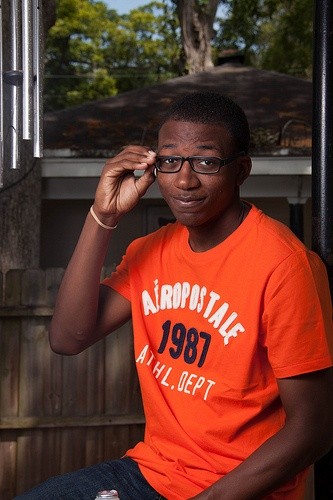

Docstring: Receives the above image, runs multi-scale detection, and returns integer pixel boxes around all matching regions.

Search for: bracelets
[90,203,119,230]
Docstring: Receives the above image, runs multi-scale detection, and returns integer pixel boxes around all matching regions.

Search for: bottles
[94,490,119,500]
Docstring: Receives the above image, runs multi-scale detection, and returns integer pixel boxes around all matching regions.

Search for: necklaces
[238,202,248,226]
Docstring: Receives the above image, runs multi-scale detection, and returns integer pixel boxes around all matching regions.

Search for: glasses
[153,152,244,176]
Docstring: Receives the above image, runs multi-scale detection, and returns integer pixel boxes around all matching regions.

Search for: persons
[7,93,332,500]
